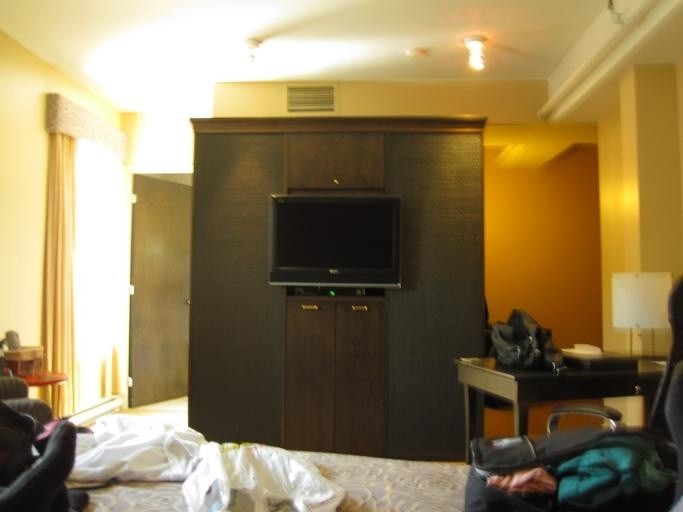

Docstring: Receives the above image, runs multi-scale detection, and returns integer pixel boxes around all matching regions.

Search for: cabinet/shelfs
[281,294,389,457]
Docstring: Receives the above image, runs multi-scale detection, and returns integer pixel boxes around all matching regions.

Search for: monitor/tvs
[267,193,402,290]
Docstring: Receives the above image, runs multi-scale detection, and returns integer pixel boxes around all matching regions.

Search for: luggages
[464,403,679,512]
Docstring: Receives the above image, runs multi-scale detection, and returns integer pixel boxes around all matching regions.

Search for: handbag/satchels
[488,308,562,370]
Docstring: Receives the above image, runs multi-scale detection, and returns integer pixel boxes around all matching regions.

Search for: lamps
[610,271,673,360]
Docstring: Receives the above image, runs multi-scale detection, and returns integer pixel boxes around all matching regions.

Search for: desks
[454,355,669,463]
[8,371,67,386]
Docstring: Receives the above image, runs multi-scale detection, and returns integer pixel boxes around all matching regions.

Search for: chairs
[546,272,683,439]
